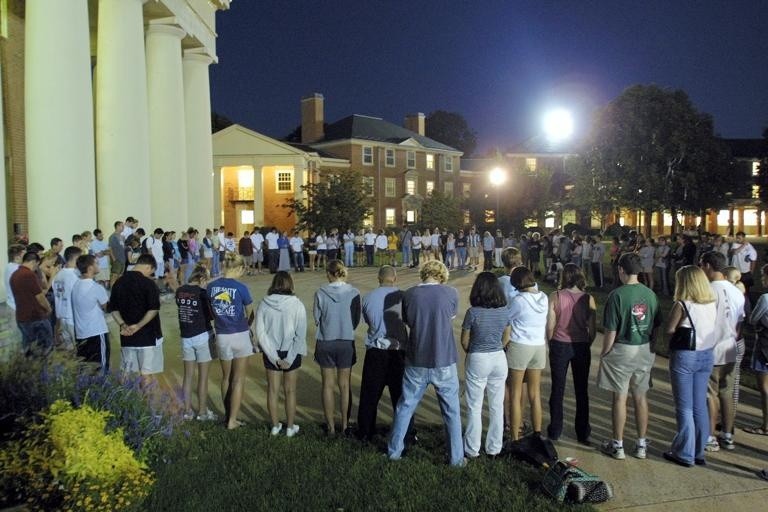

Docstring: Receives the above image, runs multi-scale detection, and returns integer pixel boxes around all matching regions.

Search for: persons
[501,267,548,441]
[459,272,512,459]
[547,263,597,447]
[387,260,468,470]
[356,264,418,445]
[700,252,736,452]
[597,253,660,459]
[251,271,306,438]
[665,264,717,467]
[8,215,767,434]
[313,260,360,436]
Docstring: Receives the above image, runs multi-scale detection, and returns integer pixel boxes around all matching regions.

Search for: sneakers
[183,412,418,462]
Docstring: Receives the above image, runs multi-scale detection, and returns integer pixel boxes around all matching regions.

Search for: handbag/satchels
[573,294,596,342]
[670,327,696,350]
